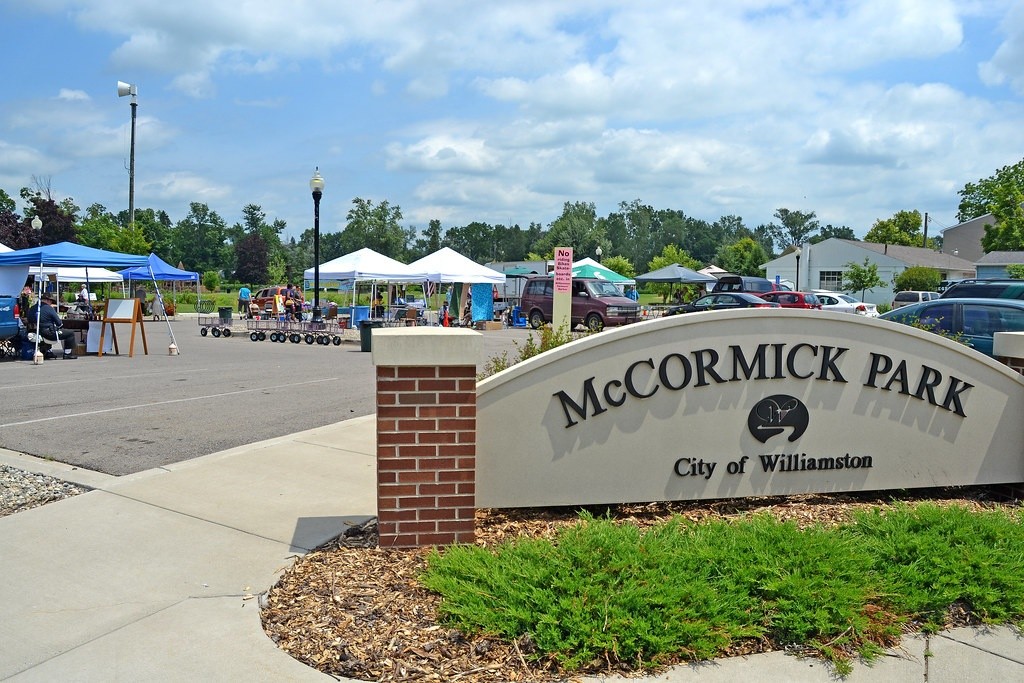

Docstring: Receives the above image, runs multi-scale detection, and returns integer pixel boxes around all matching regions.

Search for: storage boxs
[476,321,504,330]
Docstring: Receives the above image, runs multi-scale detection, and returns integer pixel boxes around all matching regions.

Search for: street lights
[30,213,42,246]
[596,243,602,263]
[116,81,138,237]
[794,245,801,291]
[310,166,323,313]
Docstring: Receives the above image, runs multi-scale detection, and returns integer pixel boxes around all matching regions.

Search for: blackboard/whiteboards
[102,298,142,323]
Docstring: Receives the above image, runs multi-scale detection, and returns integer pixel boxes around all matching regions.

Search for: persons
[21,284,32,310]
[625,285,639,301]
[674,286,706,304]
[439,300,450,327]
[152,293,164,321]
[372,295,384,317]
[59,296,68,312]
[30,292,78,359]
[136,288,147,314]
[81,284,88,299]
[237,283,251,320]
[76,294,86,302]
[286,283,305,322]
[460,302,472,327]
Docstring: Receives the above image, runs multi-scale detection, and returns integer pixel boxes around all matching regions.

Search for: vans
[249,287,304,315]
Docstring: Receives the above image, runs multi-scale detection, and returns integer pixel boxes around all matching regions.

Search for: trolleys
[248,319,345,346]
[196,316,234,337]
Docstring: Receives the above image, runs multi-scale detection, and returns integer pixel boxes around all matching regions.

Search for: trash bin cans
[359,320,382,352]
[218,306,233,325]
[512,306,526,327]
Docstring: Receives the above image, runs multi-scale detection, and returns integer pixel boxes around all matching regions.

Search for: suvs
[521,276,643,332]
[875,277,1024,365]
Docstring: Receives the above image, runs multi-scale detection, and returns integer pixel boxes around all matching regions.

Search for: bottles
[168,341,177,355]
[33,347,43,365]
[67,306,102,321]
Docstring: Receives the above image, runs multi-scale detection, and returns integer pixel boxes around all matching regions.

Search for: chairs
[27,316,58,360]
[374,306,423,328]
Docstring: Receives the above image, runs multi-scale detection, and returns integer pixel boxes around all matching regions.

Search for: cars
[663,277,880,318]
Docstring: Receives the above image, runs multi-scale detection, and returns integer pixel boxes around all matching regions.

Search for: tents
[0,242,199,364]
[632,263,718,299]
[303,247,431,328]
[548,258,636,300]
[404,246,507,325]
[698,265,730,292]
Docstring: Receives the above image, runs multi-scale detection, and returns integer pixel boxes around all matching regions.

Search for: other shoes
[240,316,242,320]
[63,352,77,359]
[46,351,57,358]
[245,317,247,320]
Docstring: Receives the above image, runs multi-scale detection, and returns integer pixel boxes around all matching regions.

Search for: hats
[81,283,86,287]
[78,294,83,297]
[37,292,56,301]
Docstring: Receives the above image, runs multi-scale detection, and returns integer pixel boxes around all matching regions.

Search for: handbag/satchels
[286,297,294,305]
